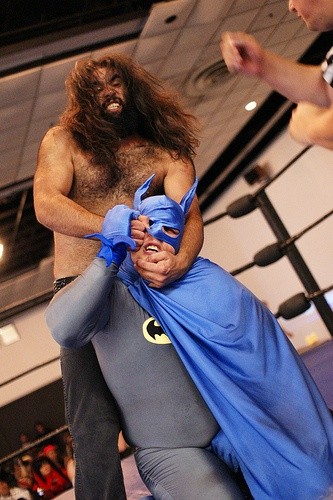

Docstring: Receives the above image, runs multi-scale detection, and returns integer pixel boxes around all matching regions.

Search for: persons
[33,54,205,500]
[220,0,333,149]
[0,423,73,500]
[45,174,333,500]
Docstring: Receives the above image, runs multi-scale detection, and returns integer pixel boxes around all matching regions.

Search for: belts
[53,275,78,293]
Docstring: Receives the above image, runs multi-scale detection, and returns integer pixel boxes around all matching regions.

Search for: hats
[43,445,56,456]
